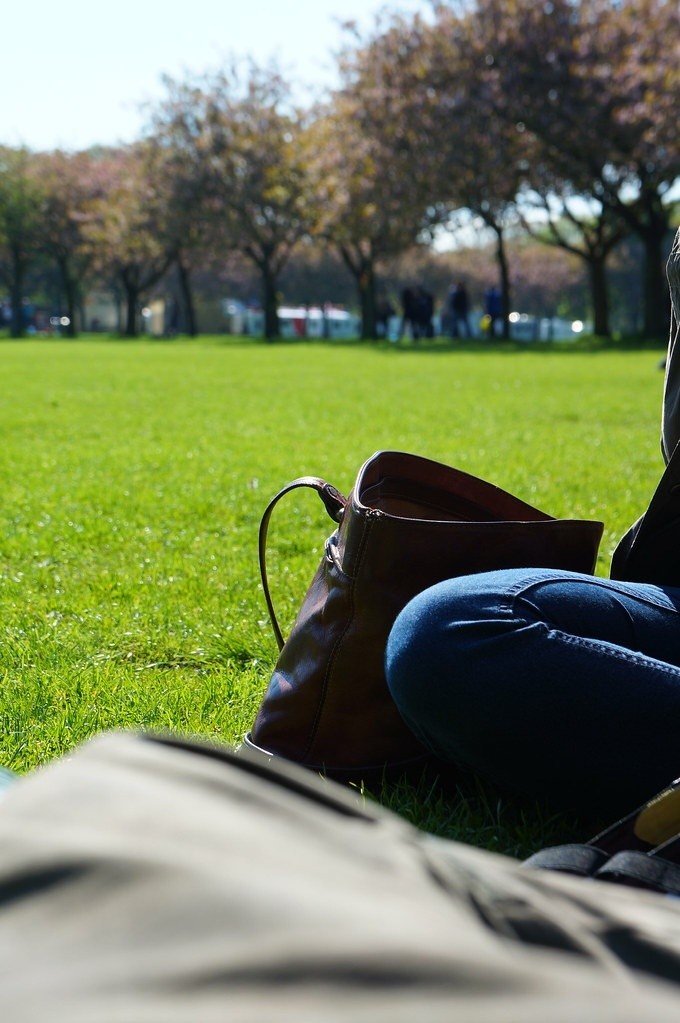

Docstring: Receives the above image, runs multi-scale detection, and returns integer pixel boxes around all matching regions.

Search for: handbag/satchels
[237,452,605,790]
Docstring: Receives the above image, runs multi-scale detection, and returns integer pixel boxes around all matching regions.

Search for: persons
[373,267,394,339]
[398,283,435,339]
[484,282,505,340]
[383,226,680,855]
[440,281,473,339]
